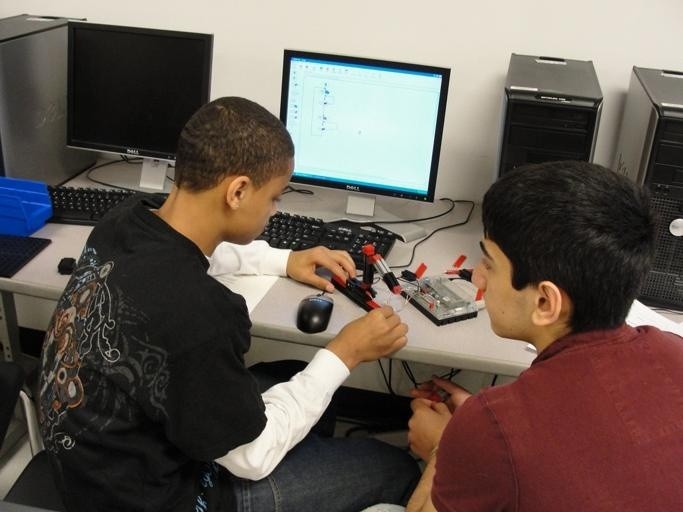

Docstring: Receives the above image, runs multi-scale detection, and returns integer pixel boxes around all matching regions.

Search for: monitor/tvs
[280,49,452,243]
[66,22,214,193]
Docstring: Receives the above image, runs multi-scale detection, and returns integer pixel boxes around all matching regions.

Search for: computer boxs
[498,52,604,181]
[611,65,683,312]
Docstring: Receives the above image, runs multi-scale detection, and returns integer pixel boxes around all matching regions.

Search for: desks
[0,151,683,494]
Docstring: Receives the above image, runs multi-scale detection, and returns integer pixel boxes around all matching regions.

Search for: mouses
[297,294,333,334]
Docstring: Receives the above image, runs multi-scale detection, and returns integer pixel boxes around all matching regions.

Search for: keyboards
[45,185,137,226]
[254,211,395,271]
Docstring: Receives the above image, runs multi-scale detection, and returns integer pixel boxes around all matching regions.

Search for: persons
[35,94,422,511]
[404,156,683,512]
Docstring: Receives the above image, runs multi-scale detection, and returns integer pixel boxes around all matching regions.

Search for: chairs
[2,358,73,510]
